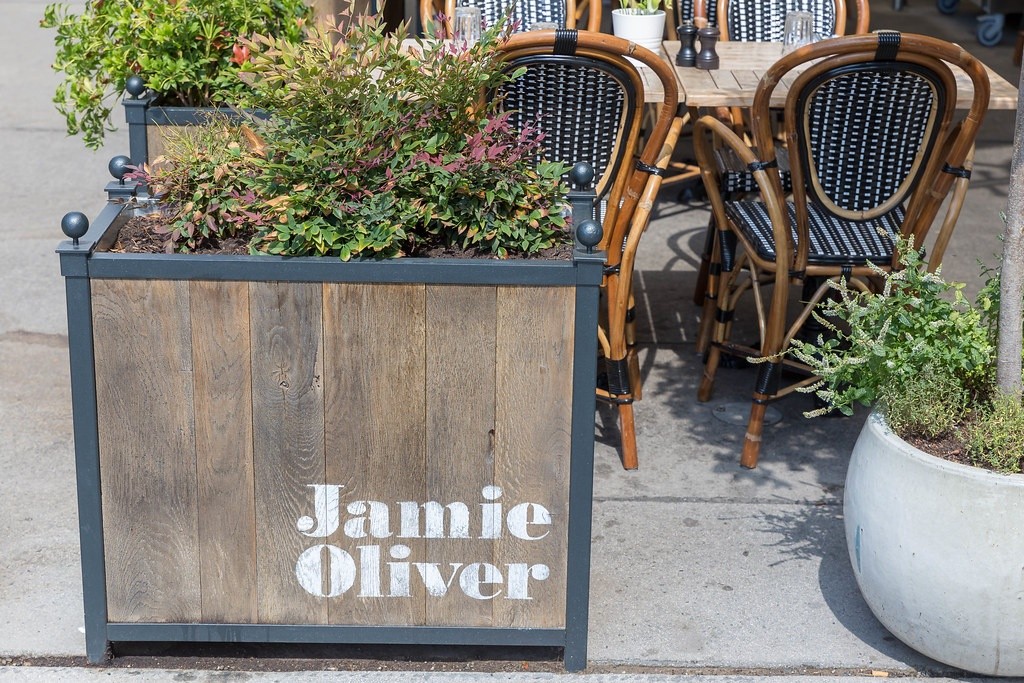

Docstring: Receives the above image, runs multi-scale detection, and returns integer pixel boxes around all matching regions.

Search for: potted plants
[56,0,608,670]
[745,45,1024,677]
[38,0,318,180]
[612,0,673,68]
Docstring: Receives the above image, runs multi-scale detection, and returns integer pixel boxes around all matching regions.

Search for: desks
[620,40,1019,351]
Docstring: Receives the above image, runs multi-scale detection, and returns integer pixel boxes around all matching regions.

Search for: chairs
[664,0,719,43]
[693,0,870,39]
[465,29,691,469]
[419,0,603,38]
[694,29,990,468]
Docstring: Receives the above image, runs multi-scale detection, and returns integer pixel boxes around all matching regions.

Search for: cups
[530,21,559,31]
[782,11,813,56]
[454,6,482,48]
